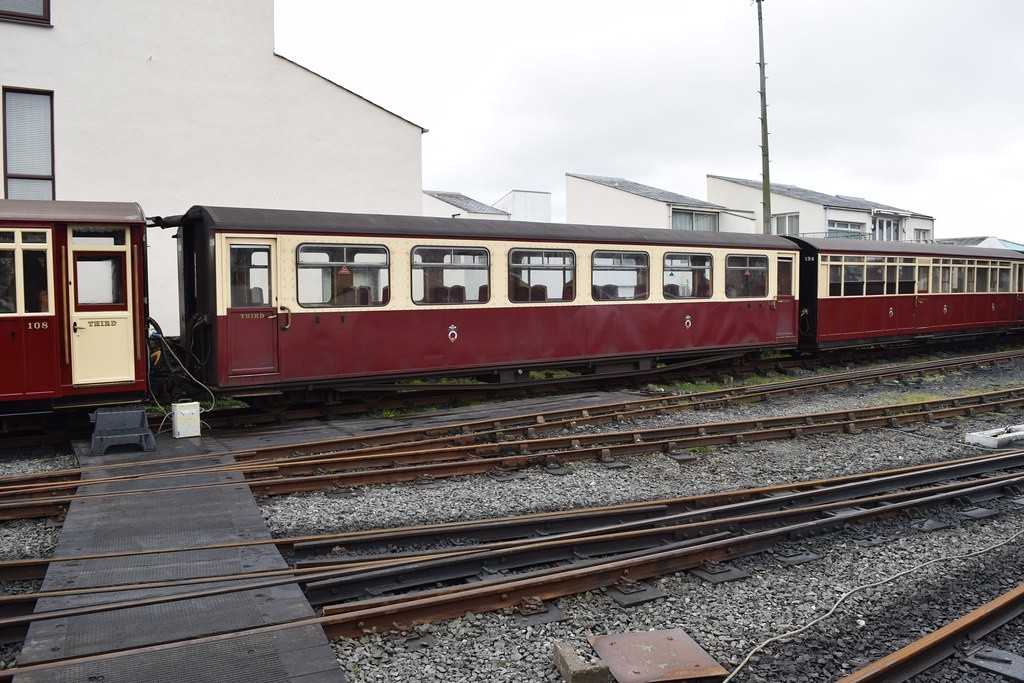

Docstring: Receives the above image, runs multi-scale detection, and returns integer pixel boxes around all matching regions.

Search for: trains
[0,197,1024,429]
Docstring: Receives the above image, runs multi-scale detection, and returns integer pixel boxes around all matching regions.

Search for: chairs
[246,278,765,307]
